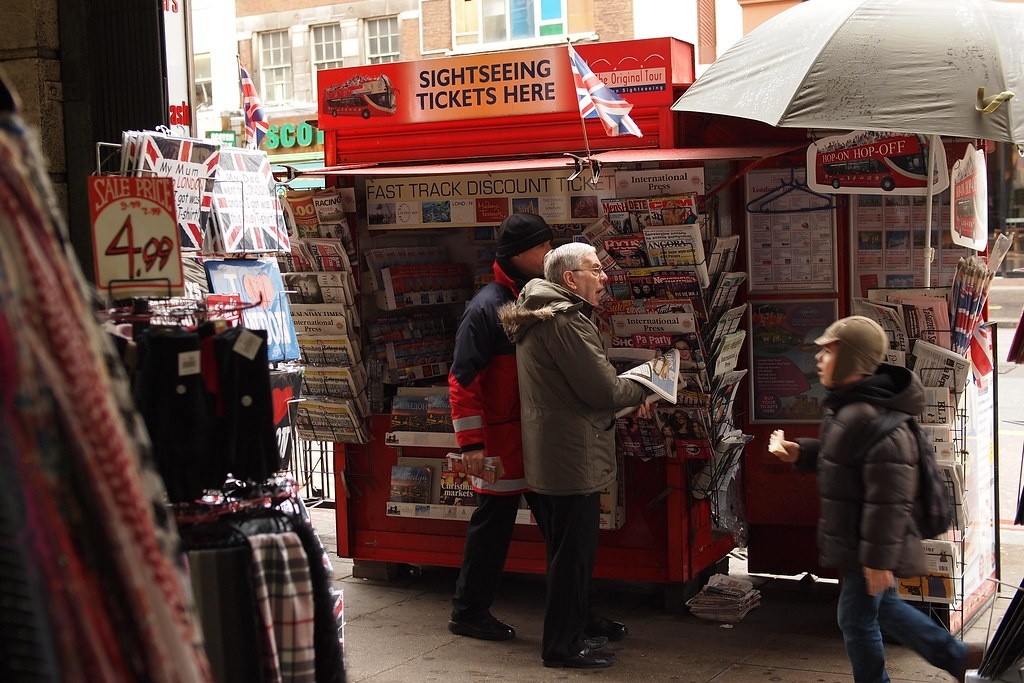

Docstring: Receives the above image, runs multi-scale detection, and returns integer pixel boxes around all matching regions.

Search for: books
[572,194,747,499]
[446,453,504,484]
[683,573,762,623]
[276,187,363,444]
[364,235,625,529]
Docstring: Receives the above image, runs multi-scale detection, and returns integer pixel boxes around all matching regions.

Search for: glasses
[569,264,602,274]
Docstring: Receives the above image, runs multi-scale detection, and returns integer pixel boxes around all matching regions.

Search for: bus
[323,72,400,118]
[815,133,939,192]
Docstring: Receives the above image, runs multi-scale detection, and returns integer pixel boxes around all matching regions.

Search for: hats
[813,316,889,382]
[496,213,553,262]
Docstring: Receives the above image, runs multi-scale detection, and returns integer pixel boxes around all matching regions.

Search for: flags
[237,61,268,150]
[567,42,643,138]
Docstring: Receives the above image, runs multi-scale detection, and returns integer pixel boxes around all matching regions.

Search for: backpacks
[853,409,951,539]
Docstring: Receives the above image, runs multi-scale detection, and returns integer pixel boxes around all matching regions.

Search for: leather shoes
[542,646,617,667]
[449,612,515,640]
[585,616,628,640]
[582,636,610,650]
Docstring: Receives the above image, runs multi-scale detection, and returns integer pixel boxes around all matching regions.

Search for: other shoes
[954,644,984,683]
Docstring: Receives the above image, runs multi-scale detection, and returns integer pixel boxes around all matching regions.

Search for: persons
[447,211,553,638]
[642,283,656,299]
[632,282,644,299]
[672,409,696,437]
[658,283,674,299]
[498,242,648,669]
[776,316,987,682]
[672,337,700,371]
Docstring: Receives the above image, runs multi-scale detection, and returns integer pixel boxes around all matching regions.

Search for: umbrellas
[668,1,1024,144]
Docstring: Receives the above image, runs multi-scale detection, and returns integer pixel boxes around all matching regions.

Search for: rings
[468,466,471,468]
